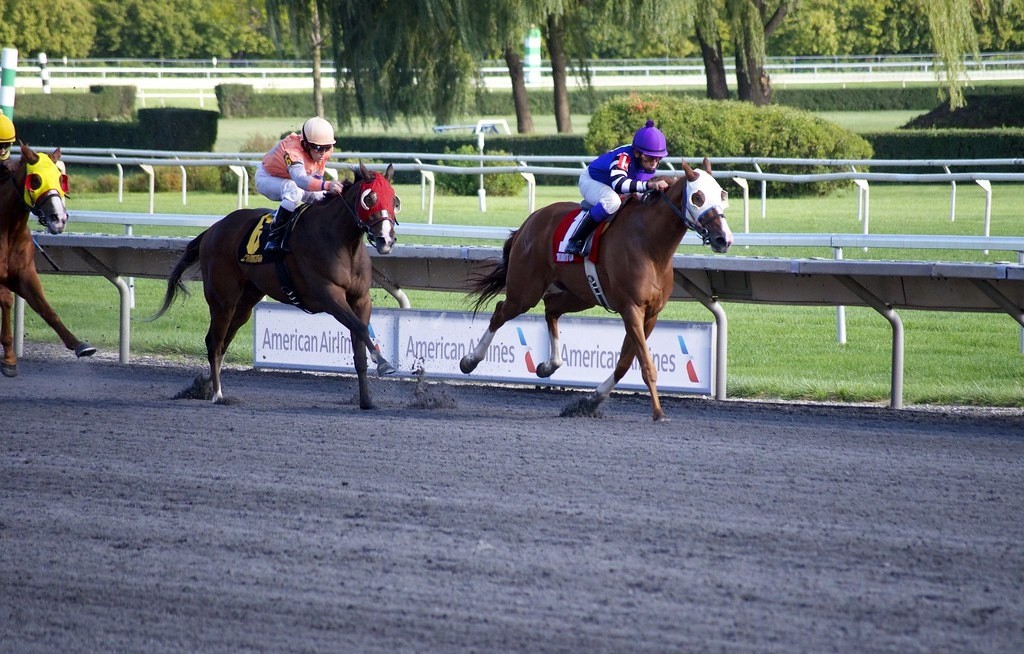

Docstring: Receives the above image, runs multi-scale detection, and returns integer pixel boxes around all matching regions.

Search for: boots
[566,210,602,254]
[263,205,293,252]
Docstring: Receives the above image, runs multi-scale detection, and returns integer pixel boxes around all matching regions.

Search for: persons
[562,120,669,258]
[1,113,16,163]
[252,116,350,259]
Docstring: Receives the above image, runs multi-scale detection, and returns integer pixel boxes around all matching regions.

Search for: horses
[147,159,398,410]
[459,156,734,421]
[0,139,96,378]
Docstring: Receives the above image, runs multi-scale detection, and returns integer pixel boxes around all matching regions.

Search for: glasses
[641,153,663,162]
[0,143,11,148]
[310,145,332,152]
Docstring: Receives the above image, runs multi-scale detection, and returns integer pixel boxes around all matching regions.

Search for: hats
[0,108,16,142]
[300,116,336,151]
[632,121,669,157]
